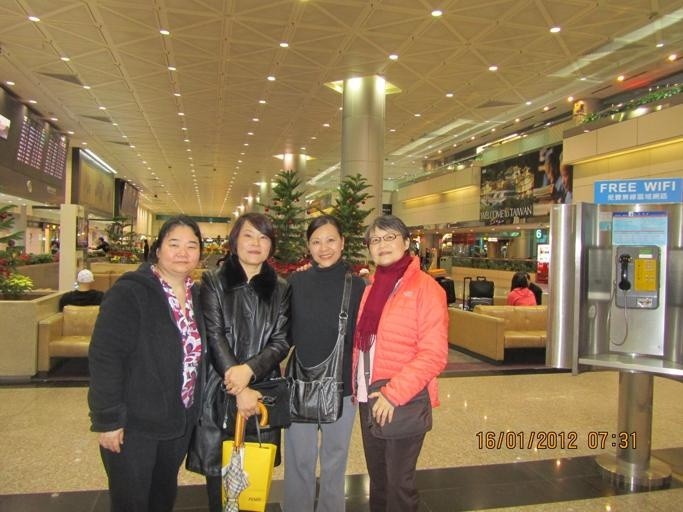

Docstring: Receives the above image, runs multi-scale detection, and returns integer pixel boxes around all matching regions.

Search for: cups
[368,233,408,245]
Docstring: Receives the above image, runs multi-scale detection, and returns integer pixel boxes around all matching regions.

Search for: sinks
[446,306,547,366]
[92,273,121,293]
[39,305,100,377]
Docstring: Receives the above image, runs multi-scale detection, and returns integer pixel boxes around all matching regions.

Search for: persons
[524,273,542,306]
[88,237,110,253]
[187,213,291,512]
[508,274,536,309]
[297,215,447,512]
[87,215,201,512]
[282,216,365,511]
[59,269,103,313]
[143,239,149,260]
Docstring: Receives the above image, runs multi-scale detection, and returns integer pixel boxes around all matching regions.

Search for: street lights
[363,348,432,438]
[216,367,293,436]
[469,276,494,297]
[285,273,351,423]
[222,409,276,511]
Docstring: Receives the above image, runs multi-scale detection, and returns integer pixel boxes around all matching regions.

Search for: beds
[222,402,266,512]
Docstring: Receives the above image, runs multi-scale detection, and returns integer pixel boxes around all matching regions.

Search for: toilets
[605,245,660,346]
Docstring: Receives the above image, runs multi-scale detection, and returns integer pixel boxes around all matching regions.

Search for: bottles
[78,269,95,283]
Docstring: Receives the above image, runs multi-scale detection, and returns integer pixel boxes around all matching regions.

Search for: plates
[441,256,536,301]
[0,293,66,384]
[89,263,140,274]
[17,260,63,292]
